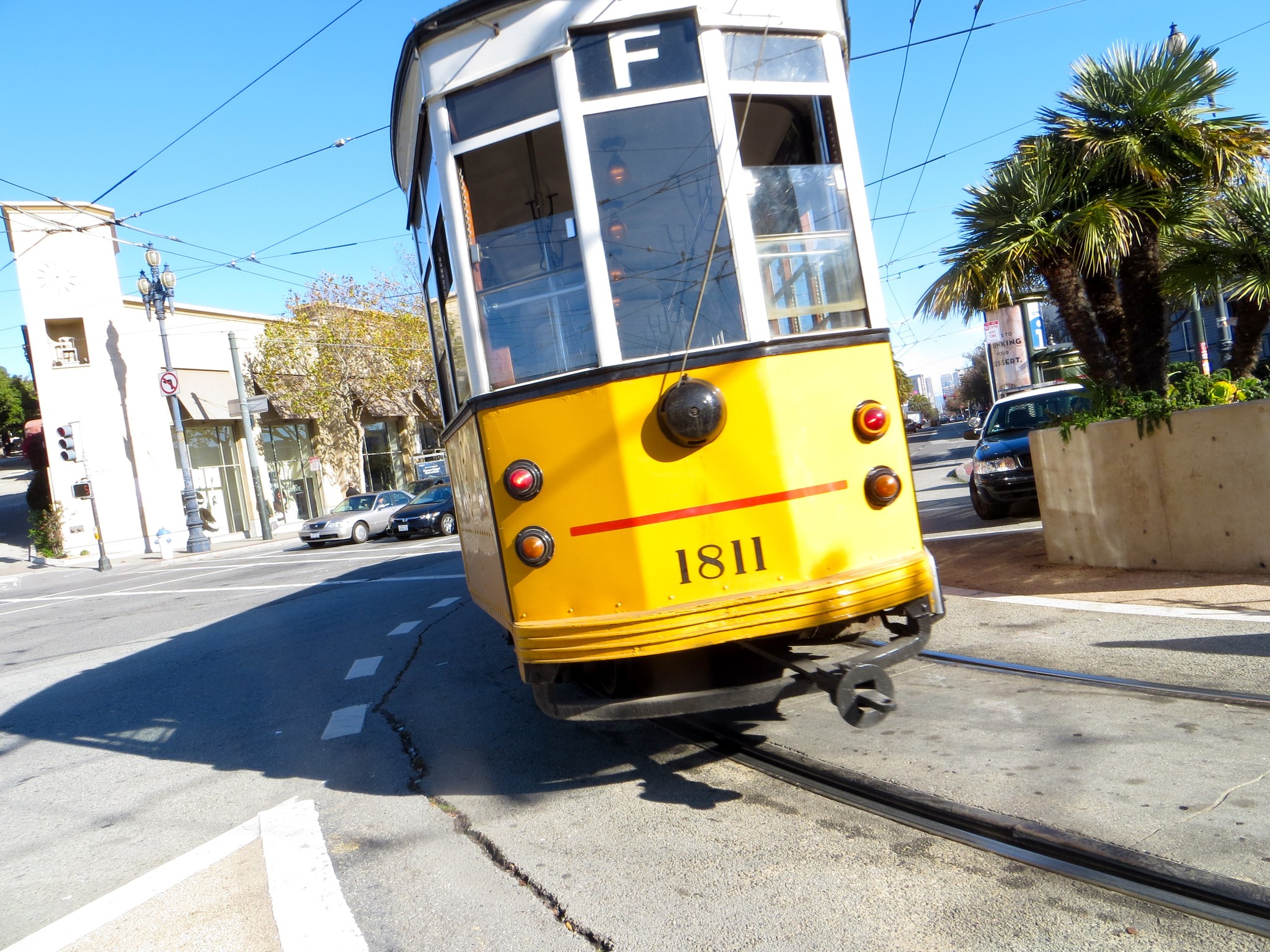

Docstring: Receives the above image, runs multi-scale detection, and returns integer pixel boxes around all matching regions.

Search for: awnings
[347,378,419,416]
[252,373,347,421]
[161,368,261,421]
[412,381,441,415]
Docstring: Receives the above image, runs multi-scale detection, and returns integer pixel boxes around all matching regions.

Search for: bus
[387,0,943,727]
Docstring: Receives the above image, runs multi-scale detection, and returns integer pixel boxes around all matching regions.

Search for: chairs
[1006,409,1036,430]
[1071,397,1088,411]
[58,336,77,362]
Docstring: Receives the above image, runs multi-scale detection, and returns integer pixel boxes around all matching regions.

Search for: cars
[401,475,449,499]
[903,419,917,434]
[938,413,965,425]
[298,491,419,548]
[386,482,458,540]
[914,422,923,429]
[966,384,1105,521]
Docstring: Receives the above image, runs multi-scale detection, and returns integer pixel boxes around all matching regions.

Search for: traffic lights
[71,482,93,498]
[954,394,957,400]
[57,423,84,463]
[944,395,947,400]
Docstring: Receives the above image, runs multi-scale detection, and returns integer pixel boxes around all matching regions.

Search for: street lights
[1161,23,1235,369]
[134,245,213,552]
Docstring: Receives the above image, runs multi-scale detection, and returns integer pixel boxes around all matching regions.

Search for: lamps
[603,201,629,243]
[602,136,627,181]
